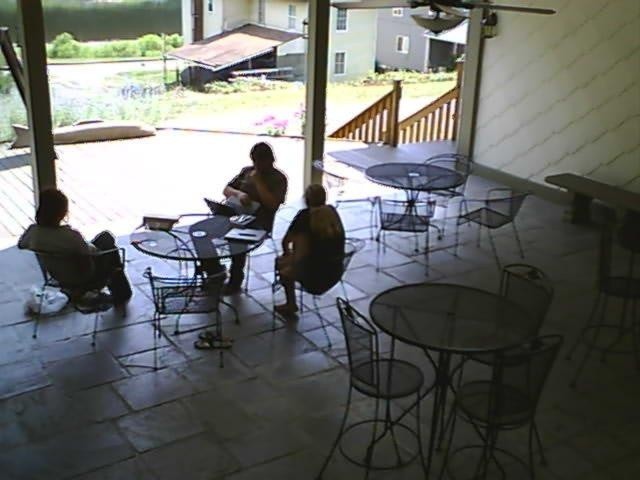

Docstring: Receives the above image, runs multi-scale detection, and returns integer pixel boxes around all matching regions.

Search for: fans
[330,0,560,24]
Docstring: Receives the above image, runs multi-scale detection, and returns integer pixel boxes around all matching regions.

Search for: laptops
[204,196,261,217]
[224,227,267,242]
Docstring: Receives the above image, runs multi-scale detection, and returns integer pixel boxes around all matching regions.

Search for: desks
[365,162,466,253]
[368,283,540,480]
[129,224,265,334]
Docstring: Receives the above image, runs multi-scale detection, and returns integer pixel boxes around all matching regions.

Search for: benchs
[543,172,639,239]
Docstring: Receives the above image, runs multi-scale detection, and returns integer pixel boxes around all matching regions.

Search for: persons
[274,184,345,316]
[189,142,288,295]
[18,188,132,308]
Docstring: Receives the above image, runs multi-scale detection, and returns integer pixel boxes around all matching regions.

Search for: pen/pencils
[239,233,256,237]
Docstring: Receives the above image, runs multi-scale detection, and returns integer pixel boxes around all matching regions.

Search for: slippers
[195,339,233,349]
[199,331,234,342]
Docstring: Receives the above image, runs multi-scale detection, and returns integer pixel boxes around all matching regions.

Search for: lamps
[408,8,469,39]
[480,13,498,42]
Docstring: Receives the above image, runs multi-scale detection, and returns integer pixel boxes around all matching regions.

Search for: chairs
[414,153,476,237]
[371,195,437,277]
[568,198,639,386]
[454,263,555,389]
[317,296,428,480]
[143,267,240,371]
[453,189,527,268]
[271,238,365,347]
[27,246,127,347]
[205,196,278,293]
[309,160,381,240]
[434,332,565,480]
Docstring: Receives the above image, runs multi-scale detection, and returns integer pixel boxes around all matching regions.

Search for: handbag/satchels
[25,285,70,315]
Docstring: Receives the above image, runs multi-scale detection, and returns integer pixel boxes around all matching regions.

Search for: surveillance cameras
[480,16,496,25]
